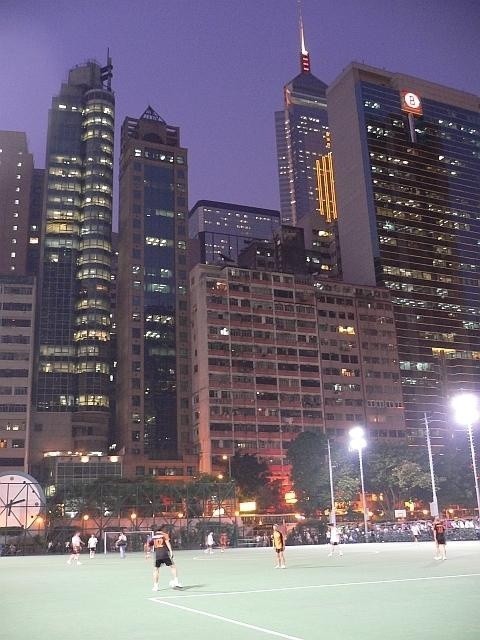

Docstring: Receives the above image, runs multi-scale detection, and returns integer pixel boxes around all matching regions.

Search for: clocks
[0,470,45,542]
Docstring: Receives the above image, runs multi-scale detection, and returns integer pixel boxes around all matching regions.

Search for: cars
[287,518,479,544]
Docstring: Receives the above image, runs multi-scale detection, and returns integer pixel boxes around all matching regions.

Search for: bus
[240,514,305,540]
[316,511,364,526]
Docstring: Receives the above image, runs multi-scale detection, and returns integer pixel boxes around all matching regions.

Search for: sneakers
[434,555,448,560]
[275,565,286,569]
[67,560,83,566]
[152,583,159,591]
[328,552,333,557]
[339,552,343,556]
[173,581,183,588]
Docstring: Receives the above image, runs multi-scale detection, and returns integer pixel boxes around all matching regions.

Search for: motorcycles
[0,544,24,556]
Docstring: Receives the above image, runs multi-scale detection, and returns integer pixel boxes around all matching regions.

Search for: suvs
[239,524,278,546]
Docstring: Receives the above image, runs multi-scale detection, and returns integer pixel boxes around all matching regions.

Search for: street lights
[223,455,232,478]
[217,474,224,522]
[234,510,239,539]
[37,517,43,535]
[84,515,89,536]
[130,513,136,531]
[451,392,480,517]
[178,513,184,531]
[348,426,370,544]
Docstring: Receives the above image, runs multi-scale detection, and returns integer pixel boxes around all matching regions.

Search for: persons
[339,523,410,544]
[208,531,214,554]
[273,524,286,569]
[256,534,274,547]
[118,532,127,558]
[147,525,183,590]
[434,518,446,559]
[293,529,330,545]
[411,517,480,542]
[88,534,98,558]
[68,532,82,565]
[328,524,343,557]
[220,532,227,552]
